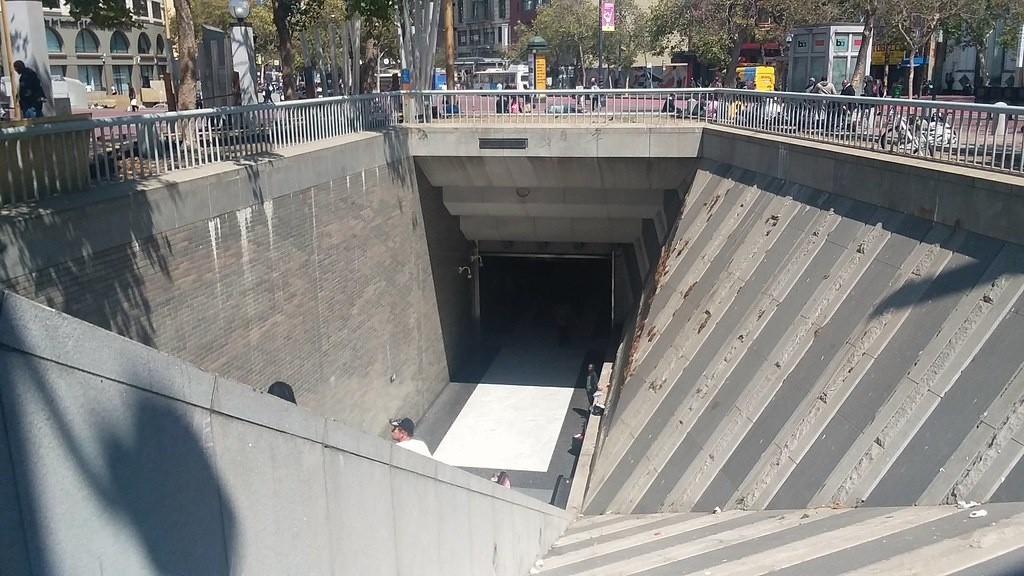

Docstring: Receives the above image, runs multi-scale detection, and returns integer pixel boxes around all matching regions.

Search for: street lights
[261,59,274,85]
[377,48,389,93]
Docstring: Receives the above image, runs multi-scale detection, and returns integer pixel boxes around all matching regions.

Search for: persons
[259,78,275,107]
[127,81,140,112]
[496,80,535,116]
[662,95,681,119]
[13,60,47,116]
[442,100,459,118]
[390,418,432,457]
[691,76,887,139]
[590,77,601,111]
[585,363,602,406]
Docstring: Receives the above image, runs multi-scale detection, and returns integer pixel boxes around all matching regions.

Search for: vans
[716,66,775,92]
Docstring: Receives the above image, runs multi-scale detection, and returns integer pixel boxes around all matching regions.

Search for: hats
[810,78,816,82]
[390,417,414,434]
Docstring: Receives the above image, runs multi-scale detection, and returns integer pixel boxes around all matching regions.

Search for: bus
[472,66,531,90]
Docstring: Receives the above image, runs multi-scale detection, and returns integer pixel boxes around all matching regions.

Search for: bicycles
[877,104,933,157]
